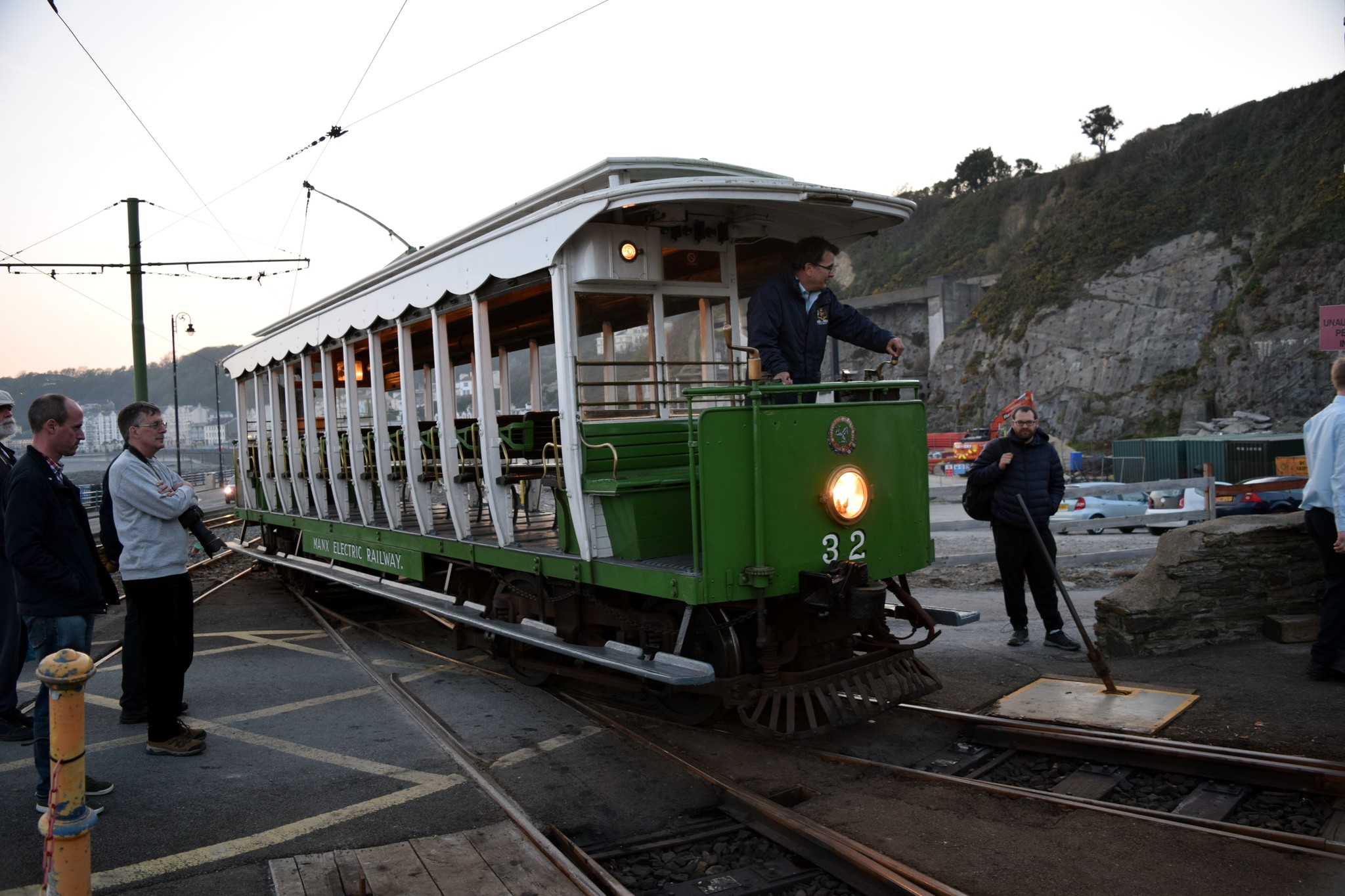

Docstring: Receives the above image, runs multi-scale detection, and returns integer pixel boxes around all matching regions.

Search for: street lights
[215,361,230,487]
[170,311,197,476]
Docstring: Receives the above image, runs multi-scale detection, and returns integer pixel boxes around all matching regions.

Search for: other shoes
[1306,650,1345,677]
[0,720,35,741]
[7,707,34,728]
[119,701,188,725]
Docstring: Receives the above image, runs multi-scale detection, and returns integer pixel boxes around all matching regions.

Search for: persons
[744,234,904,404]
[969,405,1082,651]
[0,389,36,742]
[4,392,116,816]
[99,402,206,757]
[1298,357,1345,684]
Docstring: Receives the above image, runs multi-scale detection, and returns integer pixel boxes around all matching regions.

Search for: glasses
[1013,418,1038,426]
[132,422,167,430]
[810,262,835,273]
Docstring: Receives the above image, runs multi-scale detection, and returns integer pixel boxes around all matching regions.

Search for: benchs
[249,406,697,559]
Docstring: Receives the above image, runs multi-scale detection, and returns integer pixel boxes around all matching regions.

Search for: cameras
[178,505,227,560]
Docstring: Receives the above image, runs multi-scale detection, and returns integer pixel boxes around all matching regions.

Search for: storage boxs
[1113,439,1310,484]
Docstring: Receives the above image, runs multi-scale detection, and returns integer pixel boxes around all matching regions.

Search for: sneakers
[84,774,114,795]
[36,795,104,816]
[175,719,207,740]
[1044,630,1082,650]
[146,734,207,756]
[1007,627,1029,646]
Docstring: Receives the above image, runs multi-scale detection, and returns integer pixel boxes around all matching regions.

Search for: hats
[0,389,16,406]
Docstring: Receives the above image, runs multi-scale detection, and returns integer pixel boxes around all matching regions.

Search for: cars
[223,475,236,504]
[1144,482,1233,536]
[1216,475,1309,518]
[1048,481,1151,534]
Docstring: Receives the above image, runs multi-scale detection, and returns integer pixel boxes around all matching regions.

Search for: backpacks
[961,436,1009,521]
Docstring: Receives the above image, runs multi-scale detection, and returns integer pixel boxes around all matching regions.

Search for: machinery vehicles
[927,389,1040,478]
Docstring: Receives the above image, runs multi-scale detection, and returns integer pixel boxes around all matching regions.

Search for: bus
[215,153,980,733]
